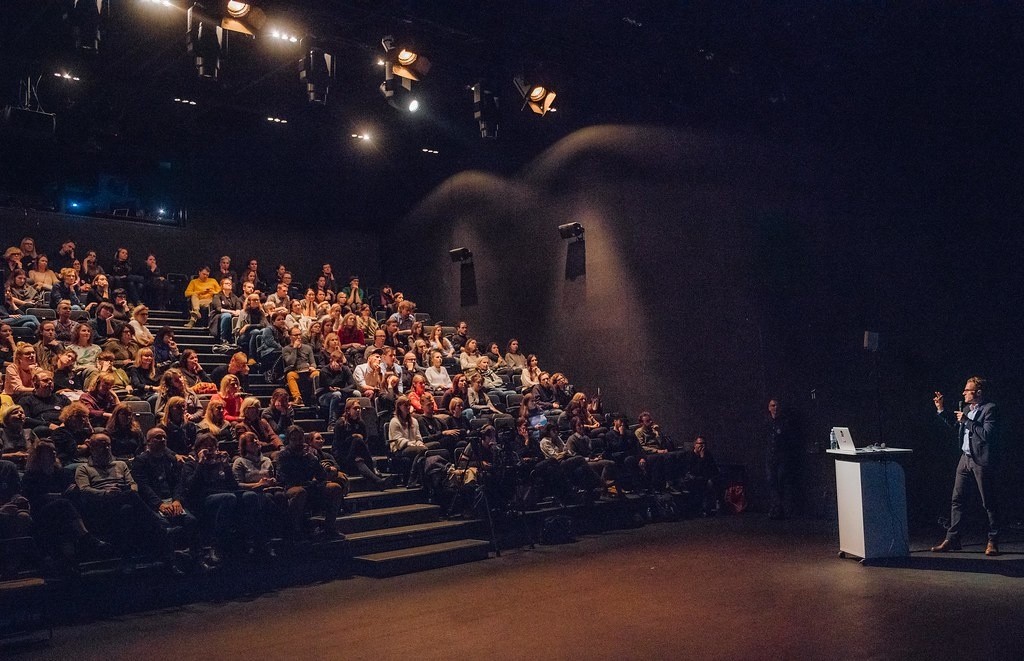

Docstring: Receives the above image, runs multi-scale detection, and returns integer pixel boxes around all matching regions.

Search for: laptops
[833,426,875,452]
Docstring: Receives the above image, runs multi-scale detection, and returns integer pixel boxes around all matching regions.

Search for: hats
[614,412,629,421]
[366,347,384,360]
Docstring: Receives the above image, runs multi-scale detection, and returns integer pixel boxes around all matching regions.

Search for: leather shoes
[986,542,999,557]
[931,539,962,553]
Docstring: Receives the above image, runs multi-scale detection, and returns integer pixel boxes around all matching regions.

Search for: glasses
[151,435,165,440]
[292,333,302,336]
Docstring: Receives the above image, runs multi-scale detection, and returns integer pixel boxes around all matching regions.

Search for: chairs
[0,240,721,652]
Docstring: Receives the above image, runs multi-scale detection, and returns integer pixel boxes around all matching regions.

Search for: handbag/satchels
[514,470,538,511]
[724,483,748,514]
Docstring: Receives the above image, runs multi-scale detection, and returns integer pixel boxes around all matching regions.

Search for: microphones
[958,401,963,425]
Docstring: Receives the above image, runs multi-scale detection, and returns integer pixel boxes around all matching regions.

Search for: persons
[762,399,793,521]
[0,237,352,580]
[184,256,724,520]
[931,376,1001,556]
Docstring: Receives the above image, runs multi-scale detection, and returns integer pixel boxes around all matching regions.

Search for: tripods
[445,435,502,558]
[490,439,536,548]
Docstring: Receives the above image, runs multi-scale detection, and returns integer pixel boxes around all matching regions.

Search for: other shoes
[202,548,224,565]
[380,477,394,492]
[81,533,110,551]
[184,321,195,327]
[264,370,274,384]
[597,479,616,487]
[326,425,335,433]
[601,494,614,502]
[288,398,306,406]
[666,486,681,495]
[321,532,347,540]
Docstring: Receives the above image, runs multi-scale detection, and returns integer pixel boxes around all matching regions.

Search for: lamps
[377,30,425,116]
[0,74,55,138]
[186,0,257,81]
[473,72,556,140]
[296,46,342,106]
[66,0,109,57]
[449,245,473,265]
[559,221,586,243]
